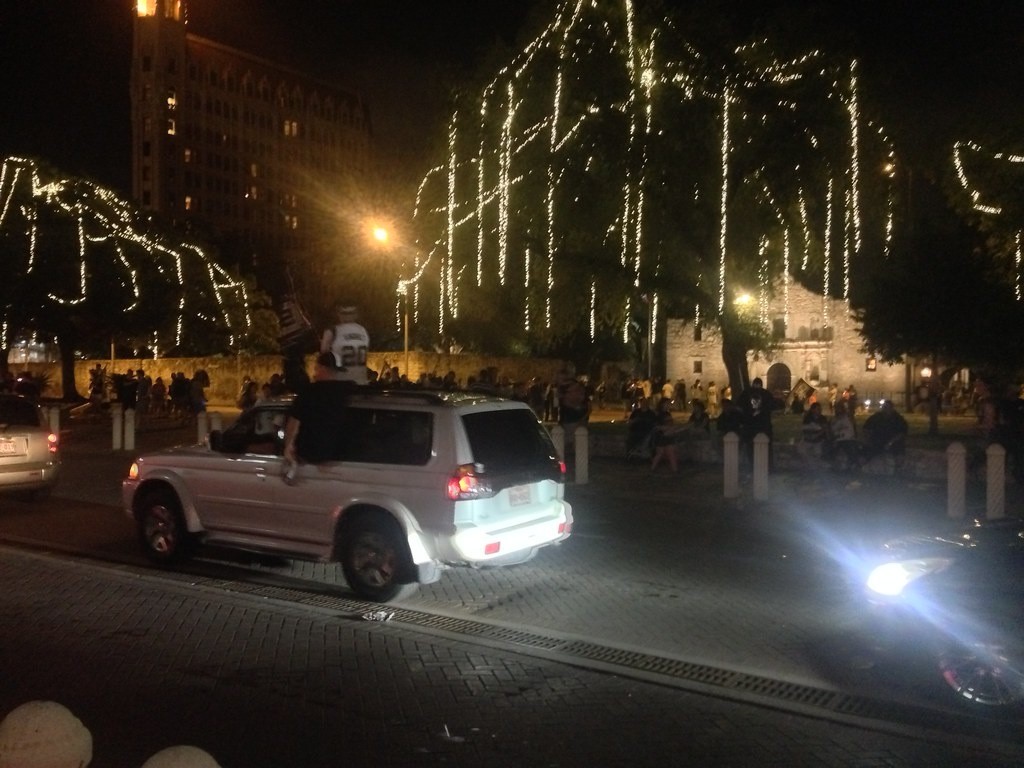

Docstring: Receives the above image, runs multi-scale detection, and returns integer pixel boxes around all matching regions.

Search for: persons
[792,383,860,415]
[715,378,774,460]
[0,370,41,397]
[238,373,283,416]
[320,304,370,384]
[971,385,1024,490]
[556,368,731,420]
[909,373,989,417]
[556,378,592,457]
[625,396,710,466]
[283,352,370,466]
[89,363,210,416]
[799,399,909,476]
[368,366,557,423]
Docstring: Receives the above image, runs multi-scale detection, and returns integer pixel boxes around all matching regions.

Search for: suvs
[121,384,574,608]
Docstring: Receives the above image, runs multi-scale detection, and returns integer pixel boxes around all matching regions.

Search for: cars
[876,517,1024,713]
[0,392,61,487]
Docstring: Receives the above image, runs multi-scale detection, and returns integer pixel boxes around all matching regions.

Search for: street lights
[373,224,411,383]
